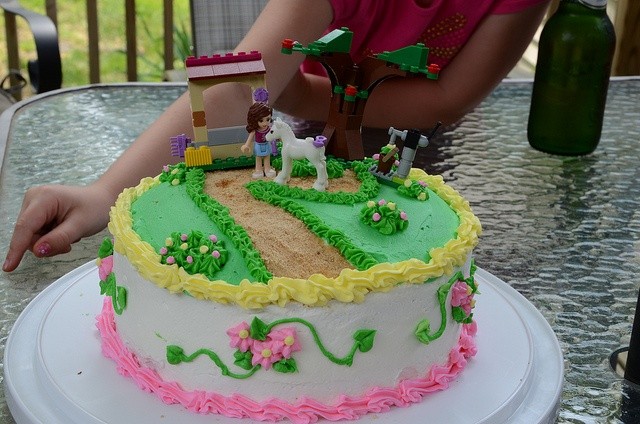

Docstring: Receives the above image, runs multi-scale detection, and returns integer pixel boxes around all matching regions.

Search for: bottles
[527,0,616,157]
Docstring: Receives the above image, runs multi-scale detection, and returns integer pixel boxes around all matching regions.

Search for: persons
[2,1,557,272]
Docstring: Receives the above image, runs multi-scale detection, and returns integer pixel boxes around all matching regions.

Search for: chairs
[190,0,271,74]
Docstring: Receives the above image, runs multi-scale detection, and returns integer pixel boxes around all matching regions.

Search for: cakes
[95,25,482,423]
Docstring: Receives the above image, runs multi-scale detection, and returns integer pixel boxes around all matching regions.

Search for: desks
[0,76,640,420]
